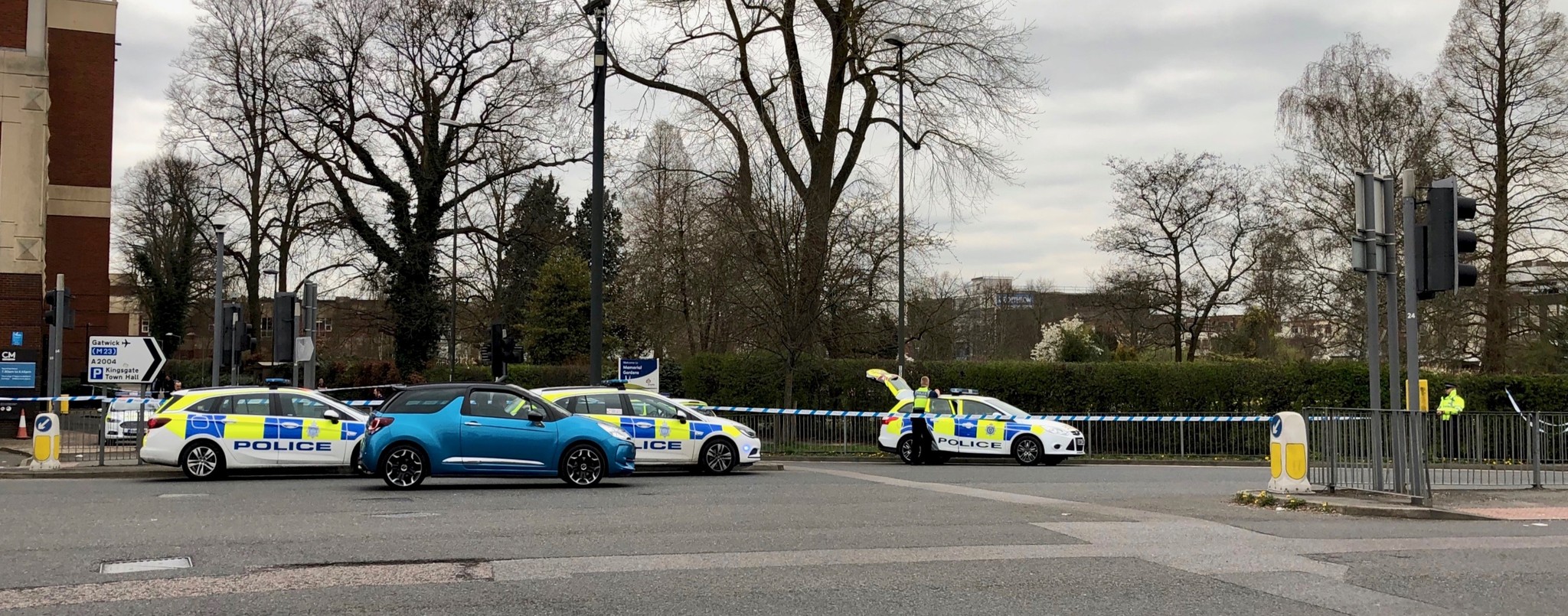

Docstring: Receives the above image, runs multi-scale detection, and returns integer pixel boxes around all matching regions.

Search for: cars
[138,379,382,479]
[565,397,717,423]
[866,367,1086,465]
[504,378,761,476]
[98,397,160,446]
[356,381,636,490]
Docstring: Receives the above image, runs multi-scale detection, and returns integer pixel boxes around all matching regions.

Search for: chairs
[475,394,493,416]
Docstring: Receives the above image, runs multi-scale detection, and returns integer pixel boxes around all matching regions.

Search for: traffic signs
[87,336,166,383]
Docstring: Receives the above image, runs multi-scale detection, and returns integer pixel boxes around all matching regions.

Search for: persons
[909,376,940,466]
[488,394,515,419]
[372,387,384,402]
[1436,382,1465,463]
[316,378,327,391]
[174,379,182,391]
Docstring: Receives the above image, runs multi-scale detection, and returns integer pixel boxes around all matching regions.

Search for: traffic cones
[14,407,32,440]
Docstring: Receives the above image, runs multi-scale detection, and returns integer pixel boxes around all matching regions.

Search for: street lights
[263,269,278,379]
[437,118,462,382]
[210,215,227,388]
[581,0,611,381]
[882,32,907,379]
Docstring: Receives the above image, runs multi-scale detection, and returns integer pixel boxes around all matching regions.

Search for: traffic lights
[234,321,257,354]
[500,335,525,364]
[44,288,76,330]
[1425,174,1478,291]
